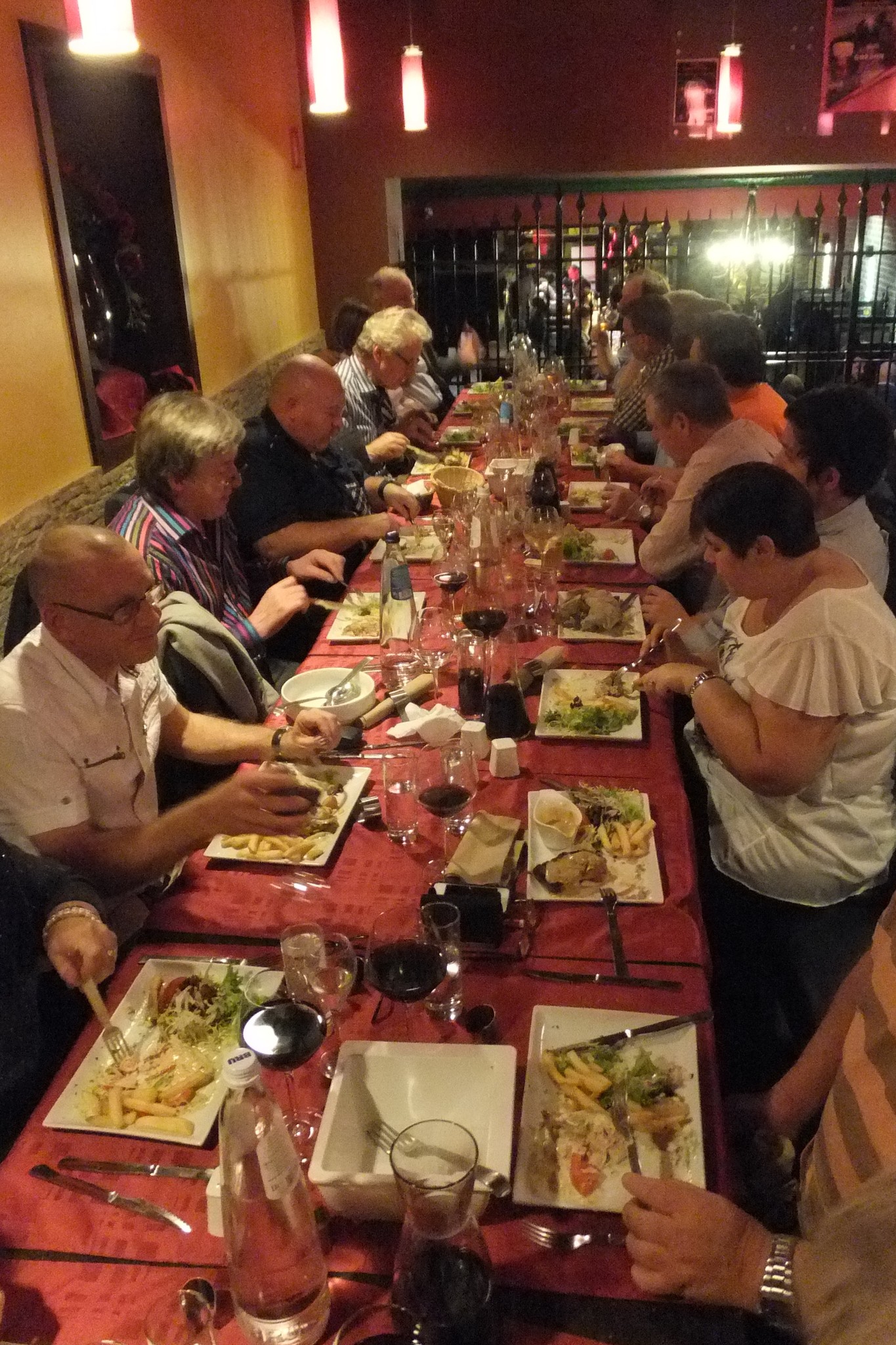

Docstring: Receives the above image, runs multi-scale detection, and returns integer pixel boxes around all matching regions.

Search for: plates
[556,587,645,643]
[40,956,285,1148]
[533,668,644,743]
[525,786,666,905]
[564,526,637,567]
[513,1002,711,1208]
[565,374,621,469]
[567,479,630,511]
[326,422,476,641]
[204,755,370,866]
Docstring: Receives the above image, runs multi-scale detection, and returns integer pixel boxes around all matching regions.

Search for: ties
[375,385,415,478]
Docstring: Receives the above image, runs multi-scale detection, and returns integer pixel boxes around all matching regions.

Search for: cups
[420,901,463,1012]
[282,921,329,1011]
[335,1302,422,1344]
[382,747,420,842]
[145,1286,215,1345]
[465,1005,502,1046]
[436,719,520,835]
[534,789,582,852]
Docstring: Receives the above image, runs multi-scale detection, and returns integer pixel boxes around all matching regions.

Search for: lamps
[308,2,354,119]
[715,0,748,135]
[396,2,429,130]
[62,2,147,62]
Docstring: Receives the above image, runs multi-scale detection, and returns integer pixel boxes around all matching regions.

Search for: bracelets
[689,669,728,704]
[40,905,103,950]
[272,724,295,764]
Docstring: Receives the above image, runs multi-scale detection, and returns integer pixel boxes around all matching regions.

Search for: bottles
[215,1049,334,1344]
[383,1117,502,1345]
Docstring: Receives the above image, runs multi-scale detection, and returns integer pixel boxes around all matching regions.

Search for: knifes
[28,1164,193,1234]
[56,1157,212,1181]
[519,964,686,995]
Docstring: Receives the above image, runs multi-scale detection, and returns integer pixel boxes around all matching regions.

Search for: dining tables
[0,373,750,1342]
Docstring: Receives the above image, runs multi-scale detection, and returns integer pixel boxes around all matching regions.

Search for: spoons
[181,1277,214,1344]
[270,681,353,717]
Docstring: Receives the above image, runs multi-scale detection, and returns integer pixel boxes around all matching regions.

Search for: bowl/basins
[309,1041,518,1226]
[282,667,375,729]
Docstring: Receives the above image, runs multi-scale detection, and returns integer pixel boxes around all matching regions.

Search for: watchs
[757,1232,801,1334]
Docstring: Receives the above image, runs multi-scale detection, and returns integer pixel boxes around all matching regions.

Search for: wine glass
[422,859,540,926]
[416,737,480,886]
[377,329,565,739]
[235,933,354,1192]
[364,906,450,1041]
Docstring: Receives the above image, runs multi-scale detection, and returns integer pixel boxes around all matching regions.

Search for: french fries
[597,816,657,857]
[541,1048,613,1119]
[78,1083,196,1136]
[221,832,333,862]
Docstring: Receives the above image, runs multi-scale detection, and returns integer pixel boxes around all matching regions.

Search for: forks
[595,883,630,978]
[396,1129,512,1200]
[609,613,683,687]
[608,1080,645,1181]
[516,1224,628,1251]
[83,974,129,1067]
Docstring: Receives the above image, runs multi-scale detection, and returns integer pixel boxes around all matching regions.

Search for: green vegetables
[544,705,632,738]
[472,376,505,393]
[562,531,595,564]
[570,780,646,823]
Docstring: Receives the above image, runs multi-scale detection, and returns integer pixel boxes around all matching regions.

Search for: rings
[107,948,118,960]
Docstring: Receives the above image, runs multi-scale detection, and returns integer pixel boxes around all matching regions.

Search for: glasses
[393,350,420,374]
[188,462,248,488]
[398,290,418,306]
[51,579,168,627]
[620,330,643,343]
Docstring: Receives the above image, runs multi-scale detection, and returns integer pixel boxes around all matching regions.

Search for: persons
[0,835,149,1165]
[623,756,896,1338]
[0,525,345,947]
[631,460,896,948]
[219,220,896,680]
[102,390,345,695]
